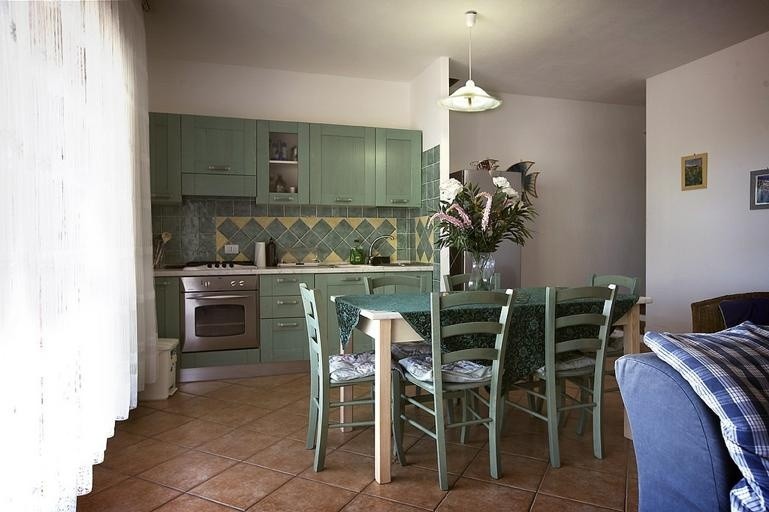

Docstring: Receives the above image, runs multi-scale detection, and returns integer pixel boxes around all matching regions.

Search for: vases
[468,252,497,293]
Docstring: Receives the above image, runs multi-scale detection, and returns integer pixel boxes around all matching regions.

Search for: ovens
[179,276,261,352]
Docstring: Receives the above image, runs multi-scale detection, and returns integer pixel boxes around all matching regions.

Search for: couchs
[692,291,769,333]
[611,319,769,512]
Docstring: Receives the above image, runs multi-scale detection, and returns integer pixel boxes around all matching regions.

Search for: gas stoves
[184,261,258,270]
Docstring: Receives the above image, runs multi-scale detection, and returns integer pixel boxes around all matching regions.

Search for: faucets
[367,234,394,263]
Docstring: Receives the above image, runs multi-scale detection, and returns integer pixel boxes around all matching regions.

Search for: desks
[329,285,654,484]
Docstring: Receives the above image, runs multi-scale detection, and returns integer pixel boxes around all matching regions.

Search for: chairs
[499,284,619,467]
[297,281,409,473]
[391,288,517,491]
[363,275,428,295]
[589,273,641,295]
[443,273,502,291]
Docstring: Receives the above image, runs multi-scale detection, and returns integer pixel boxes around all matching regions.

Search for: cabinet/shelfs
[315,274,383,355]
[180,115,258,198]
[383,273,433,296]
[154,277,181,338]
[309,123,377,206]
[150,112,181,204]
[258,120,309,206]
[376,127,421,207]
[260,273,315,362]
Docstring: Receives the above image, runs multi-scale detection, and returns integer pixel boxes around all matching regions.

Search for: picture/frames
[681,154,708,190]
[749,168,769,209]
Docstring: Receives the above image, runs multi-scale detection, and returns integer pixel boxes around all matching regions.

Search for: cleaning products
[350,240,364,264]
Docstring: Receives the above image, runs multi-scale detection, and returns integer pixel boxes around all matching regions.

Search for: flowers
[427,176,537,254]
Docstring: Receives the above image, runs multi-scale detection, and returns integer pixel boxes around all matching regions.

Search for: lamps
[437,12,502,114]
